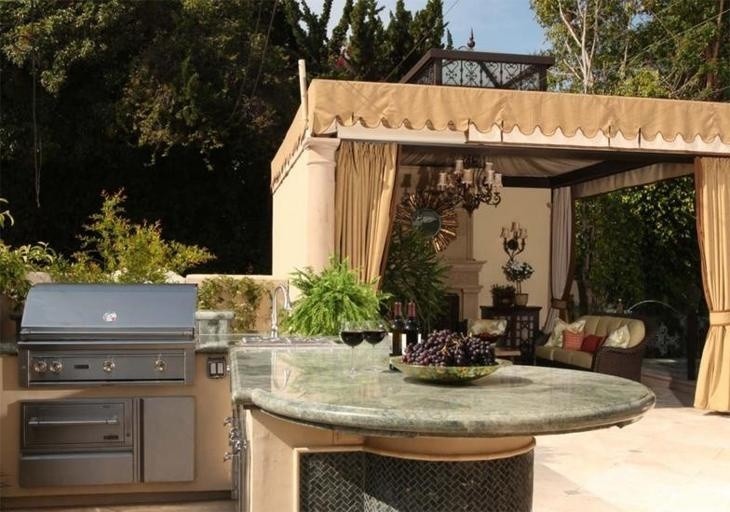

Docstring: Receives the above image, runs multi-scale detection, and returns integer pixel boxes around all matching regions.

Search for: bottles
[391,299,423,360]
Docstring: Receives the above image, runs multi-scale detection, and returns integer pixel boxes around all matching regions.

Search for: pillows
[544,316,631,354]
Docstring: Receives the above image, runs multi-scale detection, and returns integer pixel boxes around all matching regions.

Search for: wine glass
[340,319,389,379]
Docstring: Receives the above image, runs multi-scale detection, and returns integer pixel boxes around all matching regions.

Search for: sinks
[286,338,338,347]
[238,333,291,345]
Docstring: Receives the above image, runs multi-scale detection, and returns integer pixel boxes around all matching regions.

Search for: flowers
[502,261,535,293]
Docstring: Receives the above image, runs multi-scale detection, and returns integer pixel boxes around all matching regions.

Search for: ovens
[16,400,139,487]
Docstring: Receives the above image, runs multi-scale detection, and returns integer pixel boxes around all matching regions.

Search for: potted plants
[490,284,516,306]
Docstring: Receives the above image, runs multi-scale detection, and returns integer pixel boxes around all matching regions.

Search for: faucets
[270,286,292,337]
[269,348,292,391]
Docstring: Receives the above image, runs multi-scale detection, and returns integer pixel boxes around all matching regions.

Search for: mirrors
[396,192,458,252]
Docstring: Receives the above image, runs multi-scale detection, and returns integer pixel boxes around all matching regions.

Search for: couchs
[535,313,657,383]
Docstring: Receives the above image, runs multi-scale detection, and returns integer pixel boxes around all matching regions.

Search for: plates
[390,355,513,383]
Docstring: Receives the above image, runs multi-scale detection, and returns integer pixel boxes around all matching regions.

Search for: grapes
[401,329,497,366]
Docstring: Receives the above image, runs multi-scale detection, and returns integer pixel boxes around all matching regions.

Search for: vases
[514,294,528,306]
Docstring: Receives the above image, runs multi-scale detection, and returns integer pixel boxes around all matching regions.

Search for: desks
[479,306,543,364]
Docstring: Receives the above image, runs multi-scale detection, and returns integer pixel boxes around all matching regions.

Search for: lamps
[437,147,502,217]
